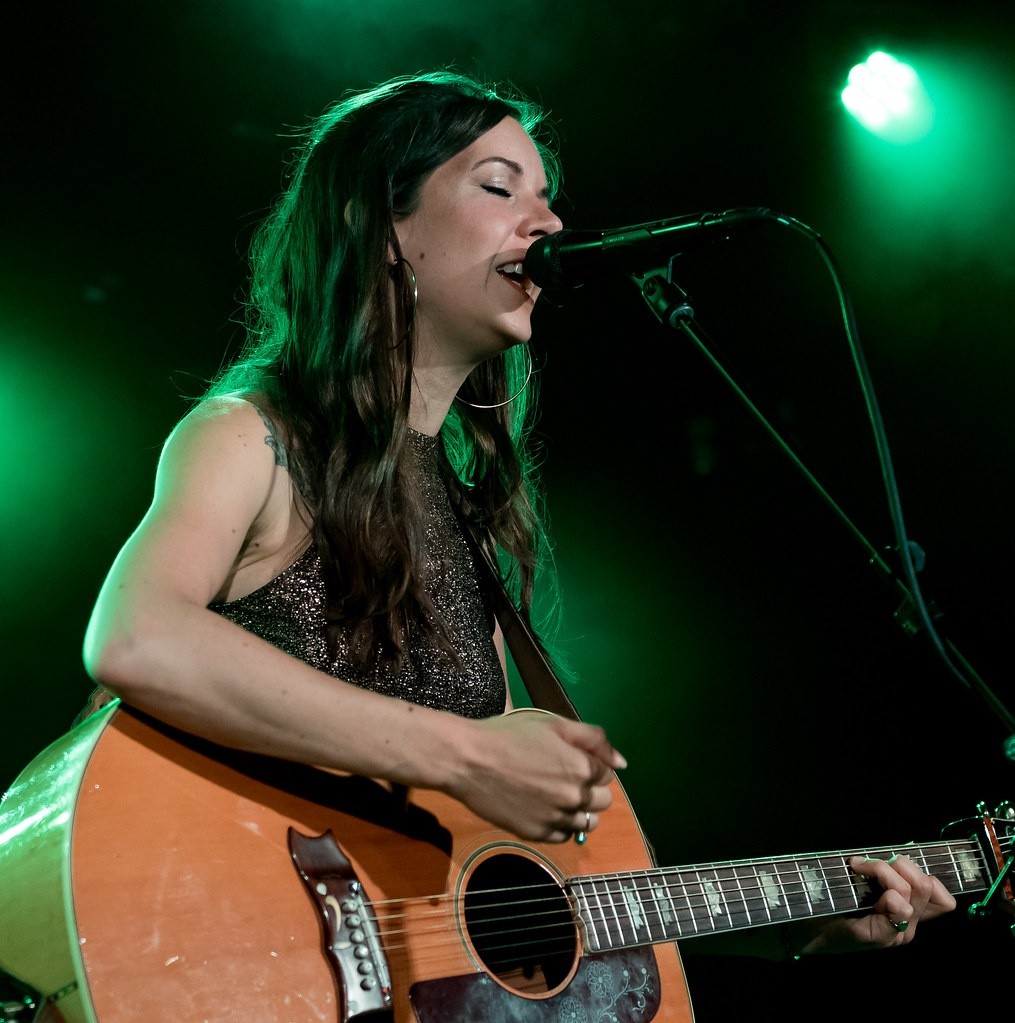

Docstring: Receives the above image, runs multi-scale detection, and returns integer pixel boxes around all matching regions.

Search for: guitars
[1,698,1015,1022]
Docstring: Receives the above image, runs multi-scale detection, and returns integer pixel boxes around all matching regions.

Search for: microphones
[524,208,771,293]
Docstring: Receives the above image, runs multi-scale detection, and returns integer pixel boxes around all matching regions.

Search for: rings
[889,918,909,931]
[575,812,590,844]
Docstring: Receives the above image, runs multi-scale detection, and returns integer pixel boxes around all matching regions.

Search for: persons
[82,72,956,1023]
